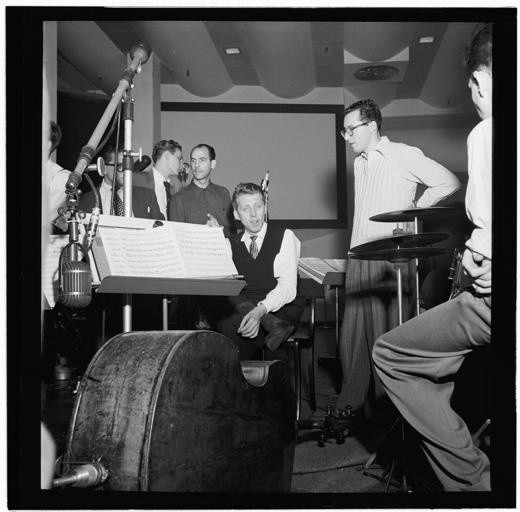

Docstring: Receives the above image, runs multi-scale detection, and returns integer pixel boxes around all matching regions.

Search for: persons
[78,146,165,371]
[311,99,462,434]
[41,121,75,417]
[372,22,492,492]
[169,144,235,238]
[132,140,184,221]
[205,182,305,360]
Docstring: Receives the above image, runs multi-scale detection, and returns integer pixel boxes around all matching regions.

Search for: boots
[236,302,296,351]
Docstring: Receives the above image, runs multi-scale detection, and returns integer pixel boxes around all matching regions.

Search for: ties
[250,236,258,258]
[111,187,123,216]
[163,182,171,221]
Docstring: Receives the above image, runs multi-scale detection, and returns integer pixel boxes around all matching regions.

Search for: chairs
[258,295,316,442]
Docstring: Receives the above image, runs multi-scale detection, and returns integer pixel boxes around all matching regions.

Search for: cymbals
[369,205,463,223]
[349,230,448,253]
[349,248,447,258]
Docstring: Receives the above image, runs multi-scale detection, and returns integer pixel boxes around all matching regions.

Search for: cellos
[61,329,359,494]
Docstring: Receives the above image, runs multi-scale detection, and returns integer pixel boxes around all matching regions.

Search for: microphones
[57,191,93,309]
[134,154,152,172]
[260,170,270,192]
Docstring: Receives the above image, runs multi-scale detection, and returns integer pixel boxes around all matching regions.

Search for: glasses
[340,121,370,137]
[104,163,123,173]
